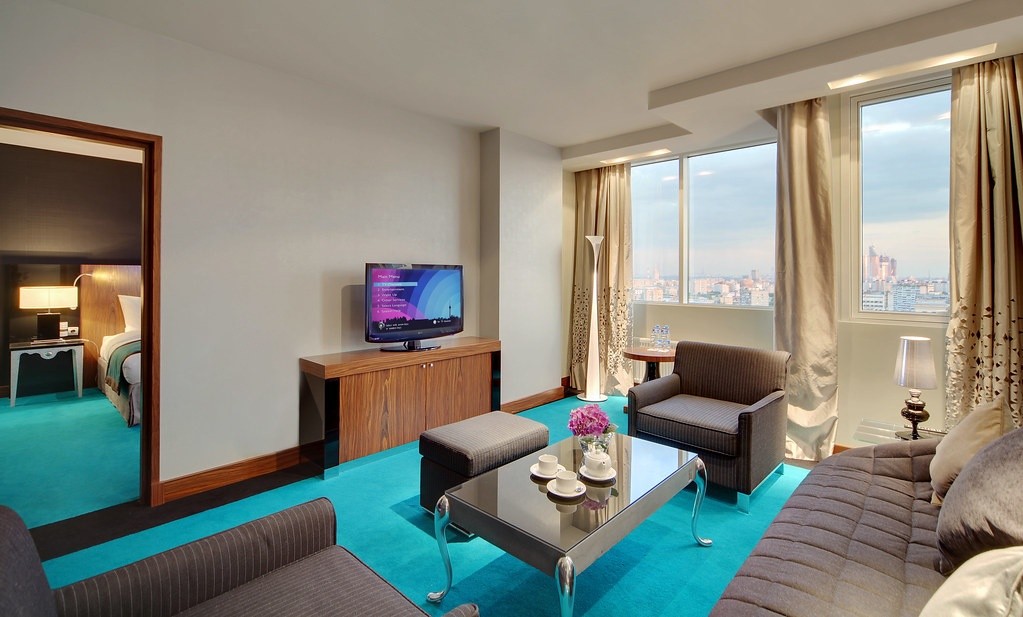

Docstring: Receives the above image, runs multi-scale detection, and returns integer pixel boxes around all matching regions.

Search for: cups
[538,484,548,493]
[556,469,577,493]
[556,503,577,514]
[538,453,558,475]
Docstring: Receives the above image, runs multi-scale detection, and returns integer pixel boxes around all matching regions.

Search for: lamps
[576,235,608,403]
[19,287,79,340]
[892,335,937,441]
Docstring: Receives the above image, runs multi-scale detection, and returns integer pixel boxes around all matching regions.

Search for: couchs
[712,436,955,617]
[0,496,479,617]
[627,340,792,516]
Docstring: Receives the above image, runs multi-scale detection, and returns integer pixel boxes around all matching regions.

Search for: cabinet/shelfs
[295,335,501,480]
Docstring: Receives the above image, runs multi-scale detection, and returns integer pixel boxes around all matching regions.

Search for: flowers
[581,489,620,511]
[568,403,619,437]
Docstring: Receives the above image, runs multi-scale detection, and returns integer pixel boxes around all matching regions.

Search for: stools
[418,410,549,538]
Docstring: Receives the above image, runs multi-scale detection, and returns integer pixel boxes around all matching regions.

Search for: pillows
[930,426,1023,576]
[117,293,142,332]
[917,544,1023,617]
[928,391,1016,507]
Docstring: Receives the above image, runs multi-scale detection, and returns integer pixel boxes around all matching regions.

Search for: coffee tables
[426,431,715,617]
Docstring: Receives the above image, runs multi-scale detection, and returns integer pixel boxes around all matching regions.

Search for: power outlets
[68,327,79,336]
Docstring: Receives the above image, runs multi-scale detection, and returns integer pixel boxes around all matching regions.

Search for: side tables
[623,346,676,414]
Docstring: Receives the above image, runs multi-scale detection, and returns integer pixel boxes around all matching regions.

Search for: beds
[80,265,142,428]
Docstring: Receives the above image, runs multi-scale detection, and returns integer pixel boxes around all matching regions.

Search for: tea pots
[584,449,611,478]
[586,485,611,507]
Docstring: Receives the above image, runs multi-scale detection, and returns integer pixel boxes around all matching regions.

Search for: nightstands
[8,339,85,406]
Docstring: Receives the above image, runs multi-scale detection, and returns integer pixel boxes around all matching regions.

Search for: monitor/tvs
[365,262,464,351]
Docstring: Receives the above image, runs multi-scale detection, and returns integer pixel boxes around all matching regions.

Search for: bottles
[652,324,671,352]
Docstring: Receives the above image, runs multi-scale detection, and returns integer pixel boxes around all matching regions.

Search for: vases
[578,433,613,454]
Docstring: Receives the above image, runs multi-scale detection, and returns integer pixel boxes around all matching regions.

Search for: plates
[580,476,616,487]
[530,462,566,479]
[530,474,556,485]
[579,465,617,482]
[547,491,586,505]
[546,479,586,498]
